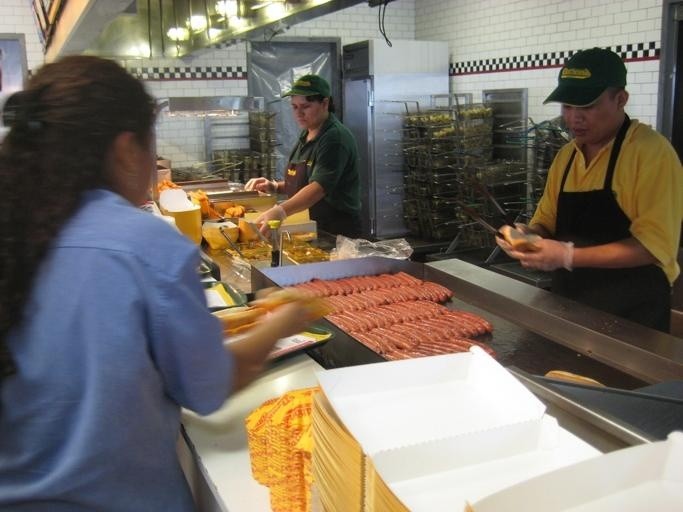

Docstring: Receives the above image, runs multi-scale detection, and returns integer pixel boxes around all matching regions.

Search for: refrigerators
[342,39,449,241]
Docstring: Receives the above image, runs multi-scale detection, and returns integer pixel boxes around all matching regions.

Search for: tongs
[456,185,516,240]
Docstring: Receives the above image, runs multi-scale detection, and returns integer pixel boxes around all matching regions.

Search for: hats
[542,48,627,108]
[281,75,331,97]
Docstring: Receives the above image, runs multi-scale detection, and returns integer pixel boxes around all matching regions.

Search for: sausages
[282,271,498,362]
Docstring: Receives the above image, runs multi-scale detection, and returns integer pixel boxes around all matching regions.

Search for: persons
[504,48,683,334]
[243,75,362,241]
[1,56,323,512]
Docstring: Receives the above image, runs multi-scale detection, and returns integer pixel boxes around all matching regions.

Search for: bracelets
[564,242,575,272]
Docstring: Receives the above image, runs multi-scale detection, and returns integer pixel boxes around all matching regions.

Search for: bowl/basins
[160,201,269,249]
